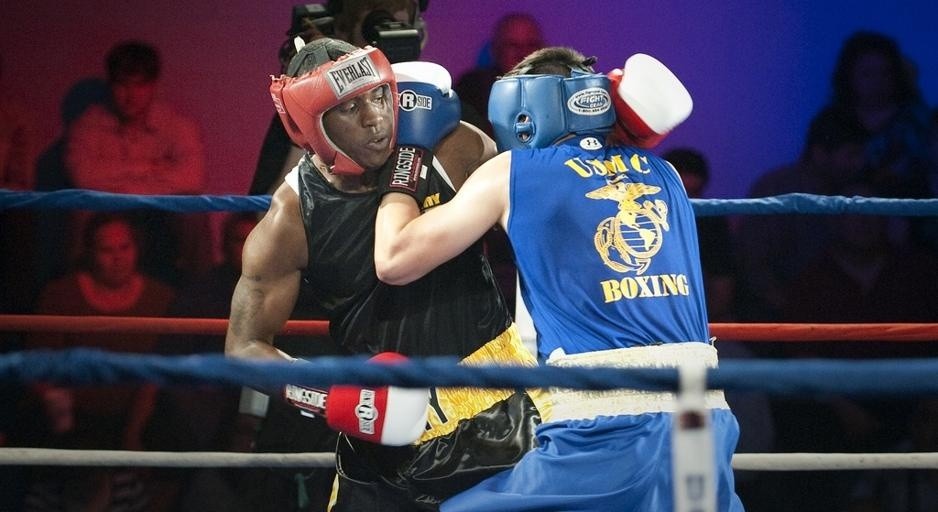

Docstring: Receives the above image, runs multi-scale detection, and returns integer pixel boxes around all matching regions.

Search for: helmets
[268,43,400,176]
[488,73,618,149]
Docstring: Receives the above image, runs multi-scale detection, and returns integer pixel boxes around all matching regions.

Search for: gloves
[606,53,695,151]
[279,352,431,447]
[378,61,464,202]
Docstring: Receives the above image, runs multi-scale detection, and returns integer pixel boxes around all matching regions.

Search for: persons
[458,12,937,512]
[0,381,334,511]
[22,213,174,452]
[64,39,208,288]
[160,213,264,453]
[224,36,693,512]
[375,46,744,512]
[250,1,429,196]
